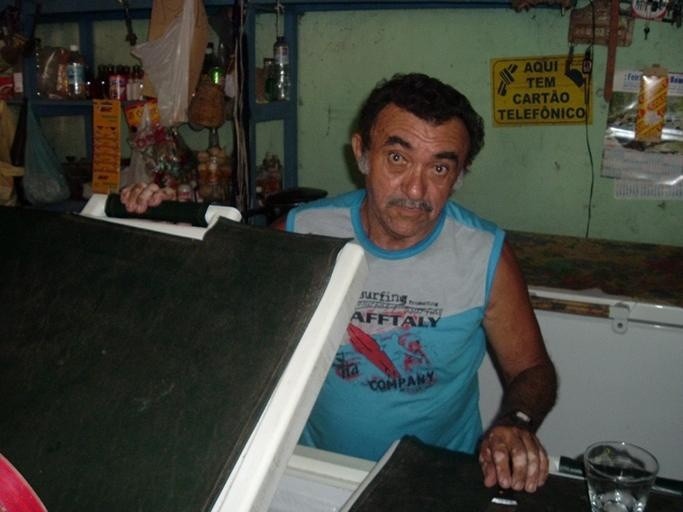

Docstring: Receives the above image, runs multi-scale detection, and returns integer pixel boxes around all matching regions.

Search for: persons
[117,71,559,494]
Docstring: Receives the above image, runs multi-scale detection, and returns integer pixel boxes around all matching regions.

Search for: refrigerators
[0,193,683,512]
[477,229,683,488]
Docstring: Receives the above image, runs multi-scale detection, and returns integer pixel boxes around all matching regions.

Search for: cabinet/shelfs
[0,1,299,227]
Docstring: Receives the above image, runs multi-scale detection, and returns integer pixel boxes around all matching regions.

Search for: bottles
[65,45,84,99]
[263,35,289,101]
[201,39,228,86]
[96,63,144,100]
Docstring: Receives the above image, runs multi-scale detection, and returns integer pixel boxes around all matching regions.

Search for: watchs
[493,407,537,436]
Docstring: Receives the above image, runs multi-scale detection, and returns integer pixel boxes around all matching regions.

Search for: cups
[584,440,660,512]
